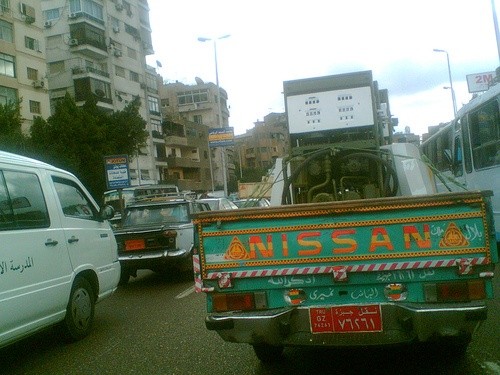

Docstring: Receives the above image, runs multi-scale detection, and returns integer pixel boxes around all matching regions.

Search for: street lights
[433,47,464,174]
[197,33,233,202]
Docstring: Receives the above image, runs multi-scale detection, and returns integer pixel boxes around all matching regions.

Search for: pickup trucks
[189,140,500,366]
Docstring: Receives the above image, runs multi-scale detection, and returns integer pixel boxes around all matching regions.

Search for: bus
[101,184,180,219]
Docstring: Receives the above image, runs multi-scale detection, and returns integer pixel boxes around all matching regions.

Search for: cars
[195,198,273,211]
[106,196,214,286]
[1,148,122,345]
